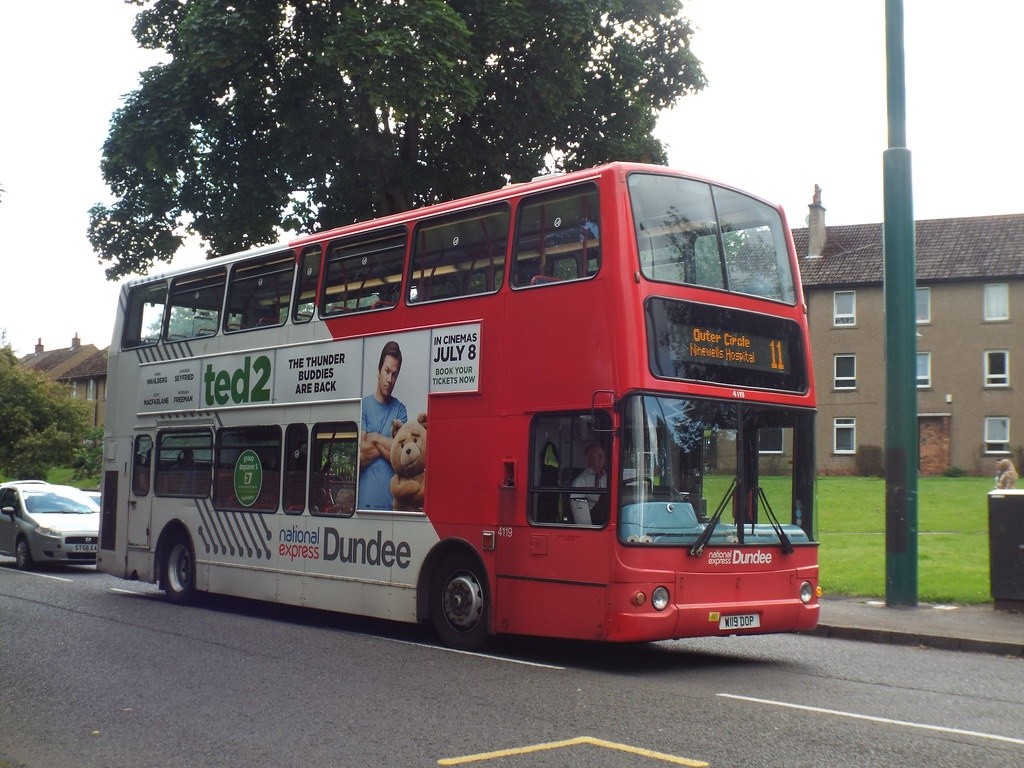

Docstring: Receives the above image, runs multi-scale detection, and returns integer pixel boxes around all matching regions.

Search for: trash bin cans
[986,489,1024,614]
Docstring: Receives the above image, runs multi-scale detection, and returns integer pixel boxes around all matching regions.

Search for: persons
[993,458,1019,489]
[356,341,408,510]
[169,450,206,496]
[569,440,610,525]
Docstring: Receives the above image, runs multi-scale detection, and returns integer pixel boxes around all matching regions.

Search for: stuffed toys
[389,414,427,511]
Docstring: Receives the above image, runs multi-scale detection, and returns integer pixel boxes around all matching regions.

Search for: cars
[0,485,100,571]
[0,480,102,508]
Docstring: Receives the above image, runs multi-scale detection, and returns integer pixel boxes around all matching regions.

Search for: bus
[96,161,822,653]
